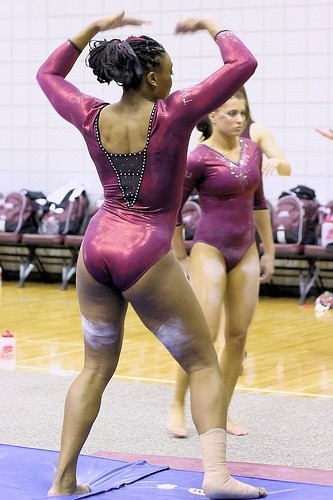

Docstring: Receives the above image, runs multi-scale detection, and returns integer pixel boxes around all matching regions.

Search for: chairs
[0,232,83,290]
[259,243,333,304]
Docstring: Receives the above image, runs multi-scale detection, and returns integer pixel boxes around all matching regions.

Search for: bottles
[1,330,16,371]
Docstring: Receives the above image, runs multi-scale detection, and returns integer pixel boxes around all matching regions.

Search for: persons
[167,91,277,436]
[200,86,291,177]
[37,10,266,499]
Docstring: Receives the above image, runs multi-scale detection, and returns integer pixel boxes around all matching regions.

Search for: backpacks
[272,186,321,246]
[38,186,89,236]
[179,190,202,240]
[0,188,37,234]
[318,199,333,251]
